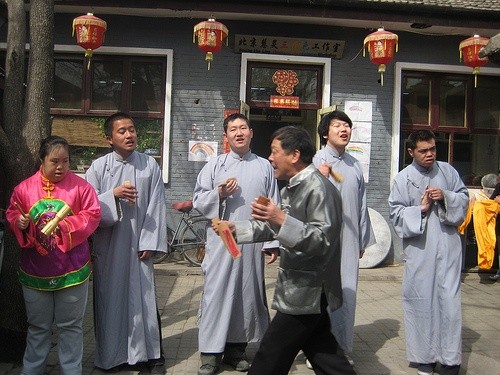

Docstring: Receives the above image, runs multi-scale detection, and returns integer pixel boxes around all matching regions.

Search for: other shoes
[489,274,500,280]
[306,359,313,368]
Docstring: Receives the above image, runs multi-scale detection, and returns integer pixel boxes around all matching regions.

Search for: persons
[85,112,168,375]
[192,113,281,374]
[387,129,470,375]
[458,170,500,281]
[212,127,357,375]
[306,111,377,370]
[5,136,101,375]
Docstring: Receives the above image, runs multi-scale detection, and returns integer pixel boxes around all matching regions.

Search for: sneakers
[418,363,437,375]
[150,363,165,375]
[222,355,249,370]
[198,364,218,375]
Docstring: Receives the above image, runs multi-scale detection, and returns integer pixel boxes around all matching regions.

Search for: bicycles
[150,200,213,267]
